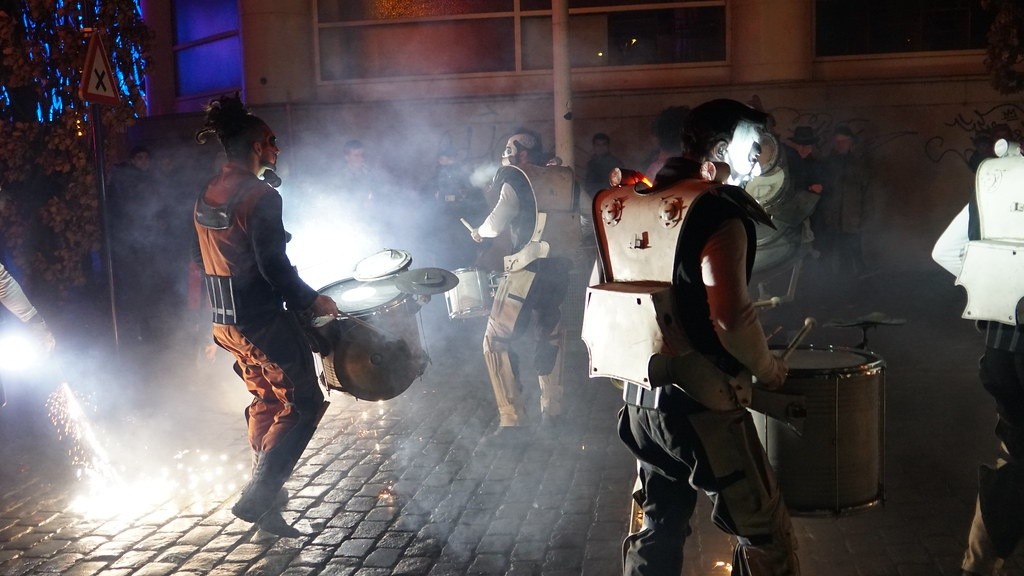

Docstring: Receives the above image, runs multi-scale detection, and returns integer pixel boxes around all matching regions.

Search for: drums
[755,131,802,176]
[352,248,412,282]
[443,267,494,321]
[314,277,433,402]
[749,347,886,517]
[750,201,807,275]
[745,163,799,209]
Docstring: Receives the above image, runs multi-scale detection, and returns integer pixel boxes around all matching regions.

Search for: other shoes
[480,426,531,449]
[241,484,290,511]
[232,488,301,538]
[542,413,561,429]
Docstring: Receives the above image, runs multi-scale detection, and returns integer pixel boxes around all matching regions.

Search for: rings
[784,371,789,376]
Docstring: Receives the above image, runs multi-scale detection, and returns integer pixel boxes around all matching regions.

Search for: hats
[788,127,821,145]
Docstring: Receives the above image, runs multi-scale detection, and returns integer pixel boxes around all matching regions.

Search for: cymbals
[394,267,458,295]
[823,318,908,327]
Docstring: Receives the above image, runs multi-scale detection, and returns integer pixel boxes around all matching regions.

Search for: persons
[322,137,497,270]
[192,85,337,539]
[539,118,870,337]
[608,100,803,576]
[107,148,214,328]
[927,136,1023,576]
[471,127,571,451]
[0,263,56,408]
